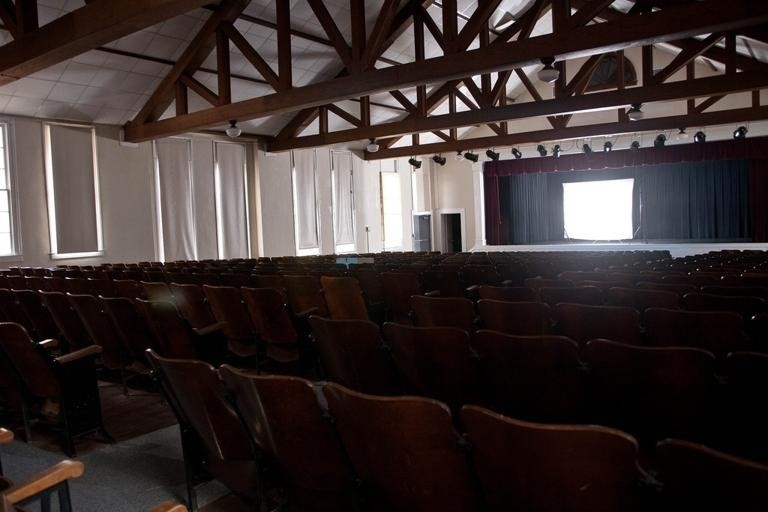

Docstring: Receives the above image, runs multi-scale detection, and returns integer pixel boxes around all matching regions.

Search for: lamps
[693,127,706,145]
[583,137,593,159]
[366,138,379,152]
[552,142,561,159]
[630,133,640,154]
[226,120,242,138]
[733,123,748,140]
[511,144,522,159]
[432,154,447,166]
[463,149,479,163]
[654,132,665,149]
[537,142,547,157]
[486,148,499,161]
[603,136,613,153]
[627,103,643,121]
[537,57,560,82]
[406,156,422,169]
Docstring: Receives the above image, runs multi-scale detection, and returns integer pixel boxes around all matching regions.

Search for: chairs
[0,251,767,512]
